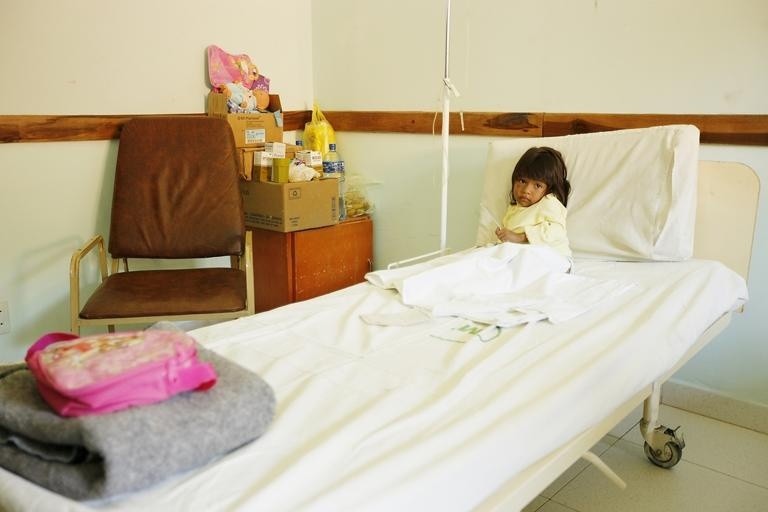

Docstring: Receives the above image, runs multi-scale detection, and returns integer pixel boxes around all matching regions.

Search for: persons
[491,144,574,275]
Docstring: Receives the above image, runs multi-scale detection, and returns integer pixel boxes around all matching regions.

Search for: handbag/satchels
[19,319,225,421]
[206,45,258,92]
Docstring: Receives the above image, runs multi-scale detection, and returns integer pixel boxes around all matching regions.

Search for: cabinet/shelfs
[252,214,371,314]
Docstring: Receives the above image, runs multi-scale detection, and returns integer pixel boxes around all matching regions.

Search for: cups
[272,158,290,183]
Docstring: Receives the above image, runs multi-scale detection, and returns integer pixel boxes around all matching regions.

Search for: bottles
[323,144,346,221]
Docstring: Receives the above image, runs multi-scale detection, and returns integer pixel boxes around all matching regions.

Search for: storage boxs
[208,93,341,232]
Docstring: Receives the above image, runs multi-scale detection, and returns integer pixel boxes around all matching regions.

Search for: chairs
[72,116,256,336]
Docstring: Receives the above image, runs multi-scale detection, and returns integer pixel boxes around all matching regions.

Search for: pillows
[476,125,700,260]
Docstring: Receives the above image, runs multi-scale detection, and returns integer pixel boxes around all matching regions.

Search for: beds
[2,160,761,512]
